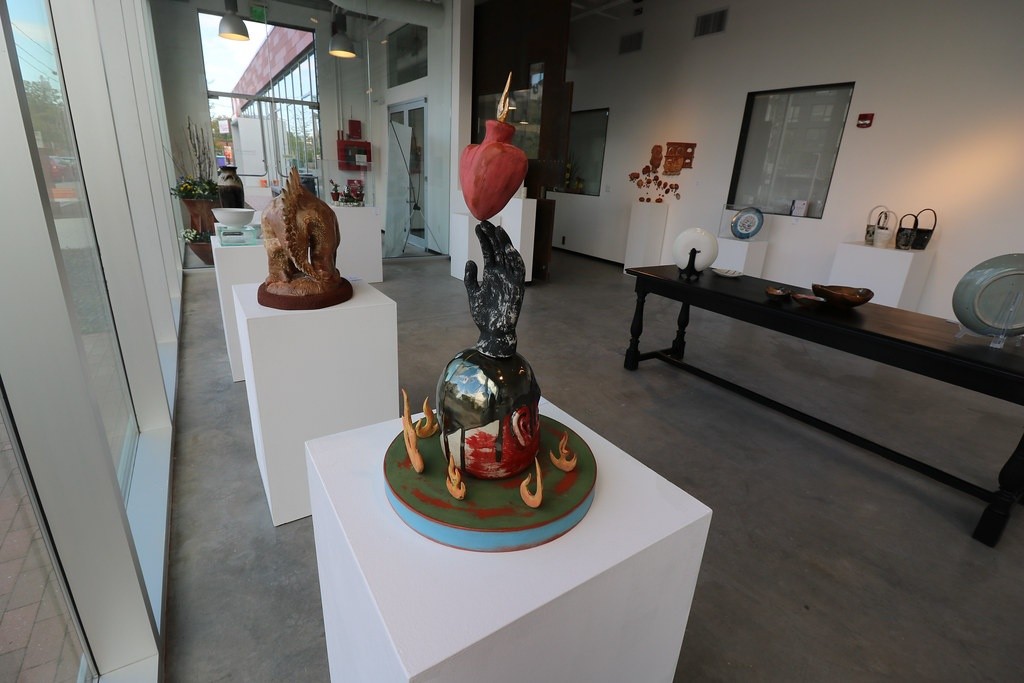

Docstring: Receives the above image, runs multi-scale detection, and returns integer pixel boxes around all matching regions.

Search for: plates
[672,227,718,271]
[952,254,1024,338]
[730,207,764,239]
[711,269,743,278]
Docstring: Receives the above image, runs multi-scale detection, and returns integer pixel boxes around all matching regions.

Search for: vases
[187,243,214,265]
[351,203,359,206]
[340,202,348,207]
[359,202,364,206]
[218,166,244,209]
[180,198,220,236]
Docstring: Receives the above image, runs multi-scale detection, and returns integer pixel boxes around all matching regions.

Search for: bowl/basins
[811,283,874,309]
[762,286,793,300]
[792,294,826,308]
[211,207,254,228]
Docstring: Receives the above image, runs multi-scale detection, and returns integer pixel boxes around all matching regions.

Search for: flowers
[163,115,218,200]
[176,229,200,242]
[341,181,364,202]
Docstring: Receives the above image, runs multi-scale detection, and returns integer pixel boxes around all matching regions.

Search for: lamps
[219,0,249,40]
[330,6,356,58]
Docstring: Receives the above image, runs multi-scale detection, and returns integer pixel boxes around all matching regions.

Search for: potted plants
[328,179,339,200]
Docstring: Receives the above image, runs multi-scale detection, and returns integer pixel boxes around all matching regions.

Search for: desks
[330,206,385,281]
[304,397,712,683]
[232,278,397,527]
[210,236,269,383]
[624,265,1024,549]
